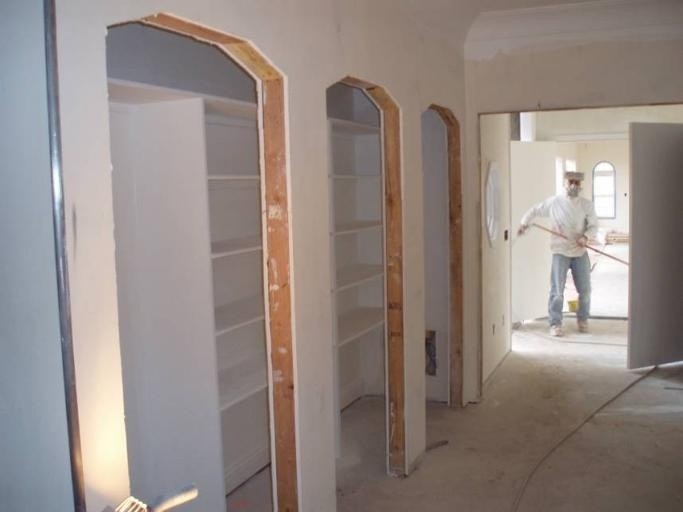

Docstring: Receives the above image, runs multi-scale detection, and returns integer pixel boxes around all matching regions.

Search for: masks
[564,184,583,197]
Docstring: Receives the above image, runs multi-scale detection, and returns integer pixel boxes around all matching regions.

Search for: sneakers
[551,325,562,336]
[578,320,588,332]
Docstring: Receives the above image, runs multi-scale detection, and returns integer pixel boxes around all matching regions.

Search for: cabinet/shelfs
[108,98,272,511]
[327,117,386,458]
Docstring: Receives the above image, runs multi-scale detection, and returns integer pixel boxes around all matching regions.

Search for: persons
[517,171,600,336]
